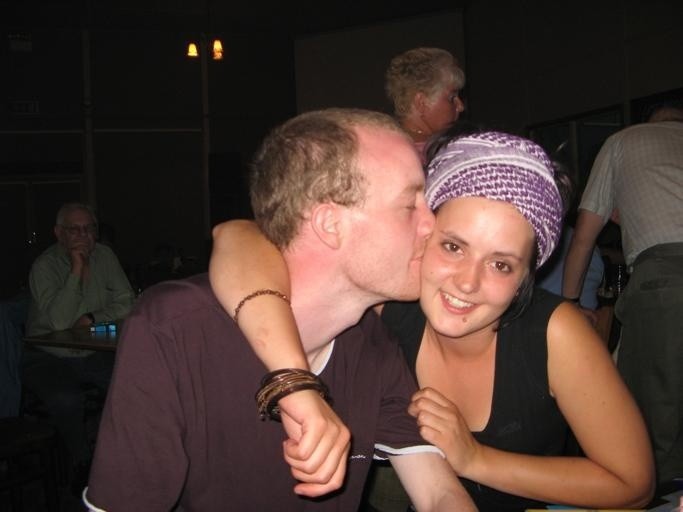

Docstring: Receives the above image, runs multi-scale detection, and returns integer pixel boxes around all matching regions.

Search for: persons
[81,106,485,510]
[17,199,136,497]
[384,46,465,176]
[539,162,604,311]
[208,128,660,510]
[558,90,683,512]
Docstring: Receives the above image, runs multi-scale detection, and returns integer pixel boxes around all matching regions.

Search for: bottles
[612,264,628,298]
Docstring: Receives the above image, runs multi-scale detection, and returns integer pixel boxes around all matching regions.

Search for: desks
[16,316,126,392]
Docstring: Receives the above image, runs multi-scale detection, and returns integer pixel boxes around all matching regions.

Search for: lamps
[186,38,224,61]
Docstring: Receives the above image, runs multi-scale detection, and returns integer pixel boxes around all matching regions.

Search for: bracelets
[83,311,96,325]
[564,295,579,306]
[252,368,336,424]
[232,288,291,329]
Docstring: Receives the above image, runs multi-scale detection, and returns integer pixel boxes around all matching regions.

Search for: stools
[1,416,61,512]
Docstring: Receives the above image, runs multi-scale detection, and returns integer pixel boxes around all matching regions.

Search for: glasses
[62,224,94,235]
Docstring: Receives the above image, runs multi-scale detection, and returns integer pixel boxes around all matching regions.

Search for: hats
[420,132,563,269]
